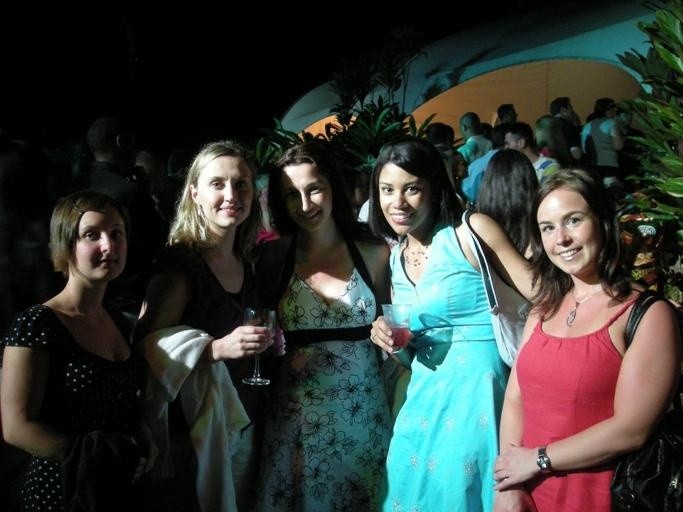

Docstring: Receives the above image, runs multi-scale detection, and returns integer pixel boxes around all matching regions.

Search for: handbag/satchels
[609,288,683,512]
[459,208,535,369]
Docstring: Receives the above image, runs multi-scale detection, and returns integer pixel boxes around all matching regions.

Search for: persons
[0,192,157,512]
[618,109,645,173]
[503,122,560,181]
[551,97,585,161]
[424,123,468,190]
[253,144,390,512]
[369,137,555,512]
[0,112,196,199]
[478,150,540,259]
[456,112,494,164]
[137,140,285,503]
[580,98,623,172]
[535,116,574,167]
[462,124,508,200]
[493,169,681,512]
[492,103,517,141]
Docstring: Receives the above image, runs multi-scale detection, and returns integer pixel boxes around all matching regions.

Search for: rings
[369,333,377,342]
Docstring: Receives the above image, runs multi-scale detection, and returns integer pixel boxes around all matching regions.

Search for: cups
[382,303,411,348]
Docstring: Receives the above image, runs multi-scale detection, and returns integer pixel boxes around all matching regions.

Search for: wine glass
[242,308,275,386]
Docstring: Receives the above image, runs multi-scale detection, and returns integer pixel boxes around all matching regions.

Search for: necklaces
[403,245,430,266]
[566,286,605,328]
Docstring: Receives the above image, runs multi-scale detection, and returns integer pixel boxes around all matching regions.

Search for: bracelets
[535,445,552,475]
